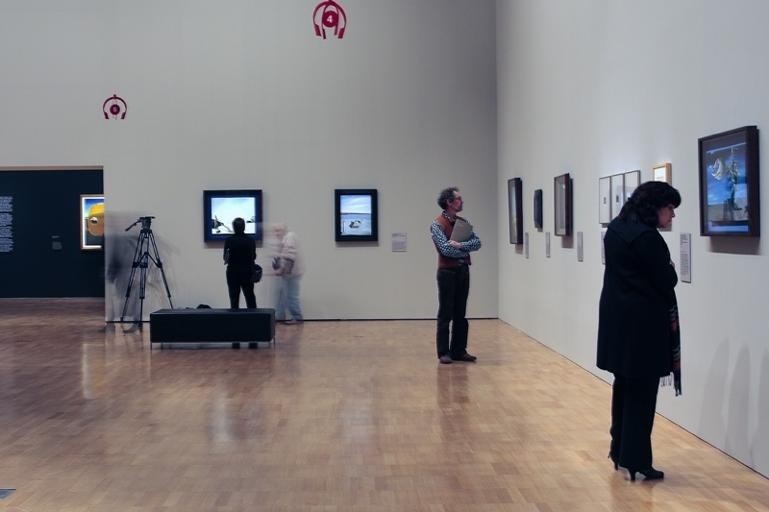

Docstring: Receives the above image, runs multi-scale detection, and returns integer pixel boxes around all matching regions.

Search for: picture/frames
[79,193,104,251]
[552,173,572,239]
[506,176,524,246]
[596,175,612,228]
[334,186,379,244]
[608,171,624,228]
[651,162,673,233]
[623,170,642,207]
[696,122,762,241]
[201,189,264,245]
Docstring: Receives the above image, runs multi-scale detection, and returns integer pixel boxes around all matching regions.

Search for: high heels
[614,459,627,472]
[627,466,665,482]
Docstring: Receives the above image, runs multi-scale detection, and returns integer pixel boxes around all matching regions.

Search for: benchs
[148,307,277,352]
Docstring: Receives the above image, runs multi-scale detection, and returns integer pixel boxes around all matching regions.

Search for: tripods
[121,216,173,328]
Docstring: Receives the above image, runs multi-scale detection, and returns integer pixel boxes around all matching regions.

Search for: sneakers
[452,352,477,363]
[232,342,259,349]
[439,355,452,365]
[285,318,304,325]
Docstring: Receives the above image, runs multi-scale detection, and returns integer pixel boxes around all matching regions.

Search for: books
[447,219,474,243]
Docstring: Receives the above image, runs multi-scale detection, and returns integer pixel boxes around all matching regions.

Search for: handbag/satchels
[248,264,263,283]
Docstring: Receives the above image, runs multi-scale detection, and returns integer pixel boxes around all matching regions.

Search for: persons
[268,221,307,326]
[222,216,262,350]
[594,181,684,484]
[428,184,484,366]
